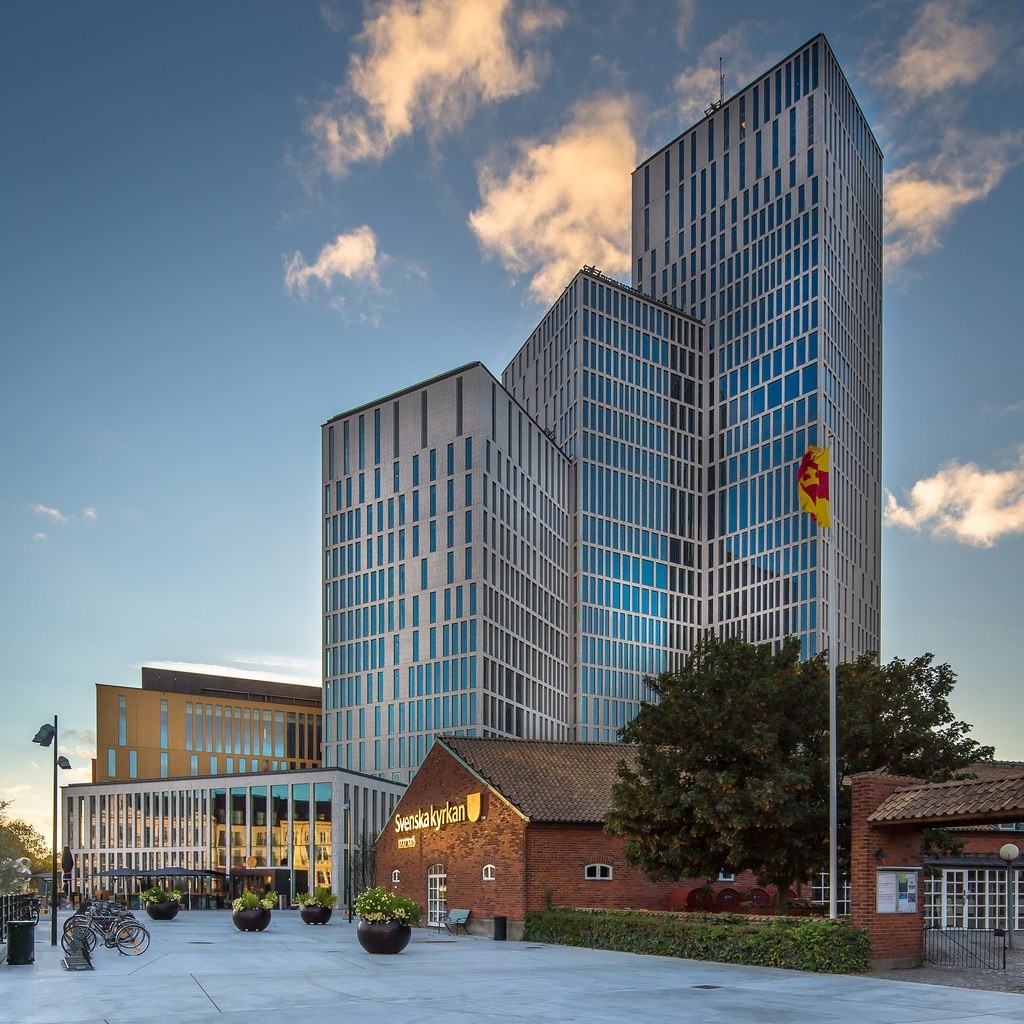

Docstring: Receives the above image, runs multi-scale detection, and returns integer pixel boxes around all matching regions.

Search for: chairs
[438,908,470,936]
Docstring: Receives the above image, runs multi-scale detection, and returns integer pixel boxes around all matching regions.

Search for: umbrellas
[189,870,229,893]
[142,867,189,892]
[62,845,73,895]
[89,868,142,893]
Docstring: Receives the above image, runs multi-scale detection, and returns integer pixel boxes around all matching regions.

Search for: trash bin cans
[6,920,35,965]
[493,916,507,941]
[278,894,287,910]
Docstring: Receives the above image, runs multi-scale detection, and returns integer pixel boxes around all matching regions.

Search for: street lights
[341,798,352,924]
[31,715,72,945]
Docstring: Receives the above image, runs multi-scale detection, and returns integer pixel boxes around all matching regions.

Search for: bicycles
[18,897,39,926]
[61,895,151,957]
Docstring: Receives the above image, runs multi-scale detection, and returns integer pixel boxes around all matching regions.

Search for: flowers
[231,890,278,912]
[352,885,423,926]
[138,887,181,903]
[294,887,338,910]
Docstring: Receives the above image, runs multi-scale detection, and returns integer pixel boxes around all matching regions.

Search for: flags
[797,446,831,528]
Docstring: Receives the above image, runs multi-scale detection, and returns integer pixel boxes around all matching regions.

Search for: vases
[357,919,412,954]
[146,901,179,920]
[300,905,332,925]
[232,908,271,931]
